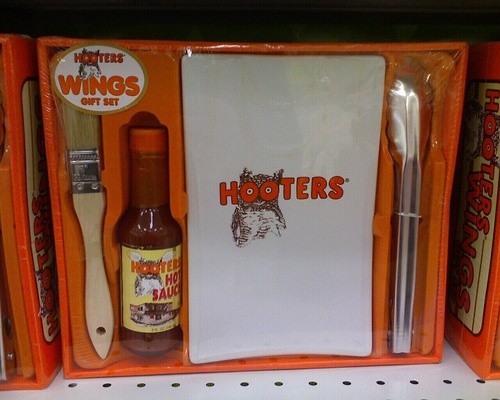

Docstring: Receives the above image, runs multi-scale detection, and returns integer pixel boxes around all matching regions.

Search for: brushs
[50,45,116,360]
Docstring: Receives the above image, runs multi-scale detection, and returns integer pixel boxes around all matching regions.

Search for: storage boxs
[33,35,471,378]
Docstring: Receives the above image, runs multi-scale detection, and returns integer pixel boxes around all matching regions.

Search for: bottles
[116,126,181,357]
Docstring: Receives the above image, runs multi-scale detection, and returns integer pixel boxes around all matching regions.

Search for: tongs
[384,55,435,355]
